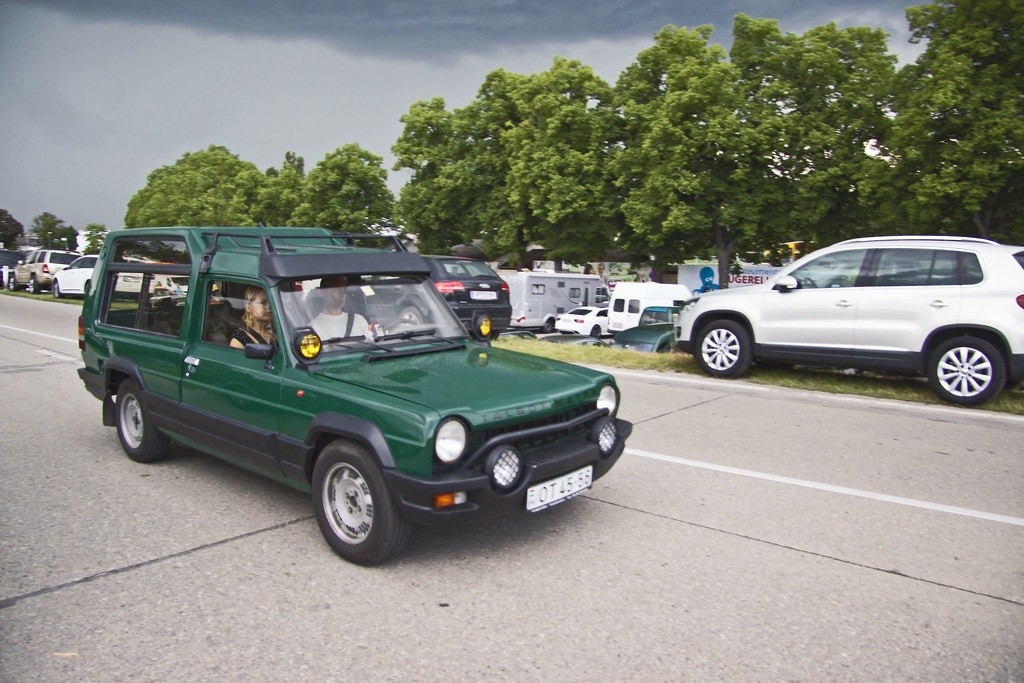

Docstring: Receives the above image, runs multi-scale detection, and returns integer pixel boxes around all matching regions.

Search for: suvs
[674,234,1023,407]
[77,224,633,568]
[307,253,513,343]
[8,249,86,295]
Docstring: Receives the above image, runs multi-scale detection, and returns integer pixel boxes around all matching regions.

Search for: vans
[638,305,681,327]
[607,281,693,333]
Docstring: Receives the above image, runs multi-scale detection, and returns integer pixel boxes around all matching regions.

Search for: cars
[610,323,680,352]
[554,305,610,339]
[155,274,191,296]
[51,253,157,299]
[539,334,611,350]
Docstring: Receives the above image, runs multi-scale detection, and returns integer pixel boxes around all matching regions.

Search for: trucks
[497,271,613,334]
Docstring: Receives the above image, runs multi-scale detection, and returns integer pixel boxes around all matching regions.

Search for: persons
[305,275,374,342]
[229,284,279,352]
[598,262,606,277]
